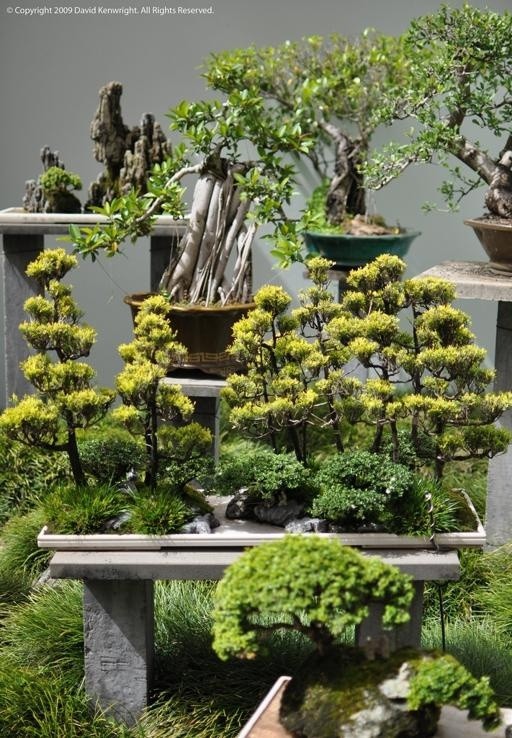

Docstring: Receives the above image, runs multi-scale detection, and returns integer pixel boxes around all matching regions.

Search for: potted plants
[356,2,512,277]
[166,36,422,272]
[60,103,257,376]
